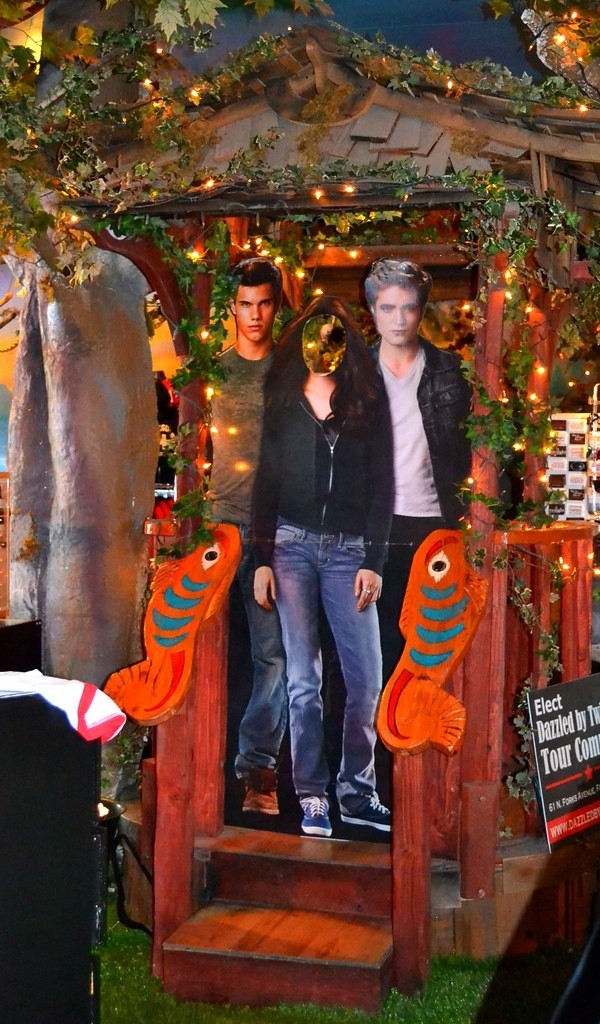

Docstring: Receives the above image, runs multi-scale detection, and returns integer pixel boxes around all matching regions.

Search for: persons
[252,296,393,839]
[211,256,282,813]
[364,261,472,678]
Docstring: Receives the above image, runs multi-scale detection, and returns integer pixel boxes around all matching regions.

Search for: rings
[363,588,373,594]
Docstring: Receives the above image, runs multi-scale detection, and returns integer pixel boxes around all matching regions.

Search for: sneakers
[341,800,393,832]
[242,769,280,816]
[300,797,333,836]
